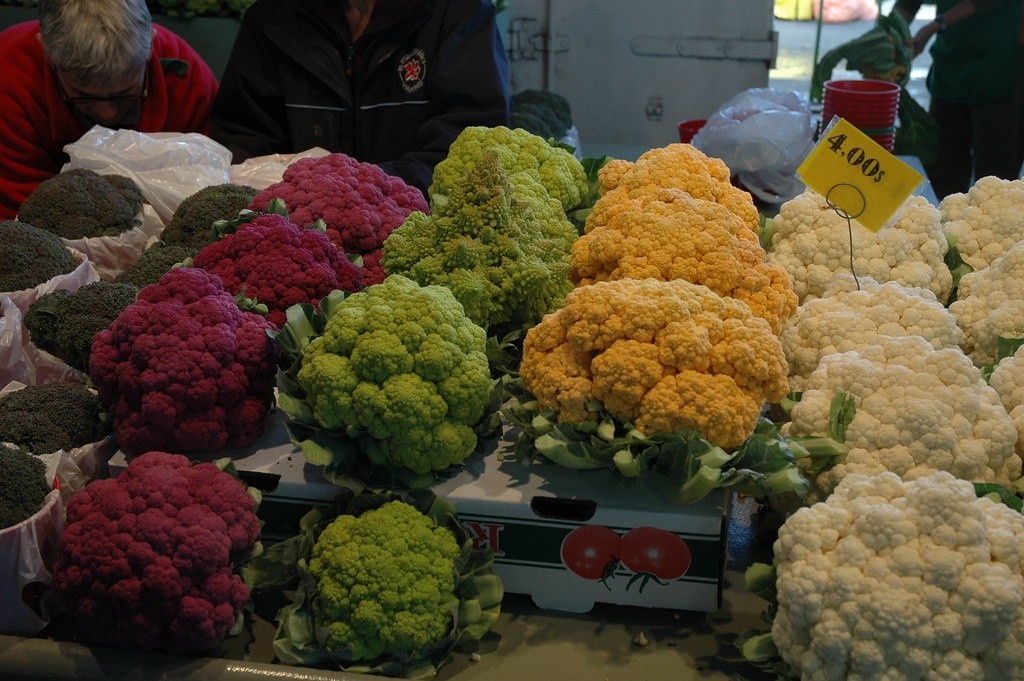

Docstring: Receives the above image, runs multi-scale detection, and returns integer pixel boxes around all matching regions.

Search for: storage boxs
[107,441,731,612]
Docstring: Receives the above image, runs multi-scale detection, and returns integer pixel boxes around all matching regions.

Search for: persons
[0,0,221,222]
[890,0,1024,207]
[204,0,510,211]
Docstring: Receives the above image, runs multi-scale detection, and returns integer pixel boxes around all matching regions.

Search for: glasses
[51,59,149,105]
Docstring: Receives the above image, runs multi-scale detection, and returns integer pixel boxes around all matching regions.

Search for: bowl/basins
[818,79,901,159]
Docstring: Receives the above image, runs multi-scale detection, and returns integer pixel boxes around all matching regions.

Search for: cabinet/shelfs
[221,144,942,681]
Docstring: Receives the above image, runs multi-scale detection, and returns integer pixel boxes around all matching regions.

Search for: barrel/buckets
[679,118,707,143]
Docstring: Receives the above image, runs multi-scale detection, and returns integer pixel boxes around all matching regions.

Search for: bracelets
[934,14,946,33]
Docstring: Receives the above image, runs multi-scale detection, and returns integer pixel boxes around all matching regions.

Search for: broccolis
[0,150,504,679]
[286,87,799,493]
[760,174,1024,681]
